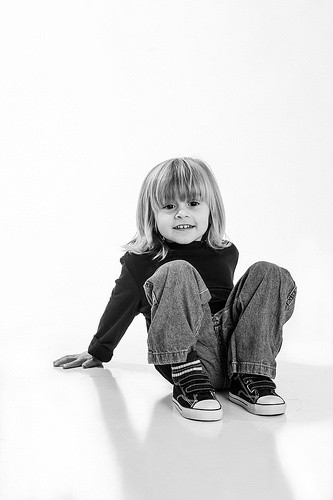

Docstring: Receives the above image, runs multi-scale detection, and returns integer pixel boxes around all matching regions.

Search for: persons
[47,157,298,423]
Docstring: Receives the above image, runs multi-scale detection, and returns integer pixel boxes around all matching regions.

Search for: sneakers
[228,372,287,416]
[172,373,223,422]
